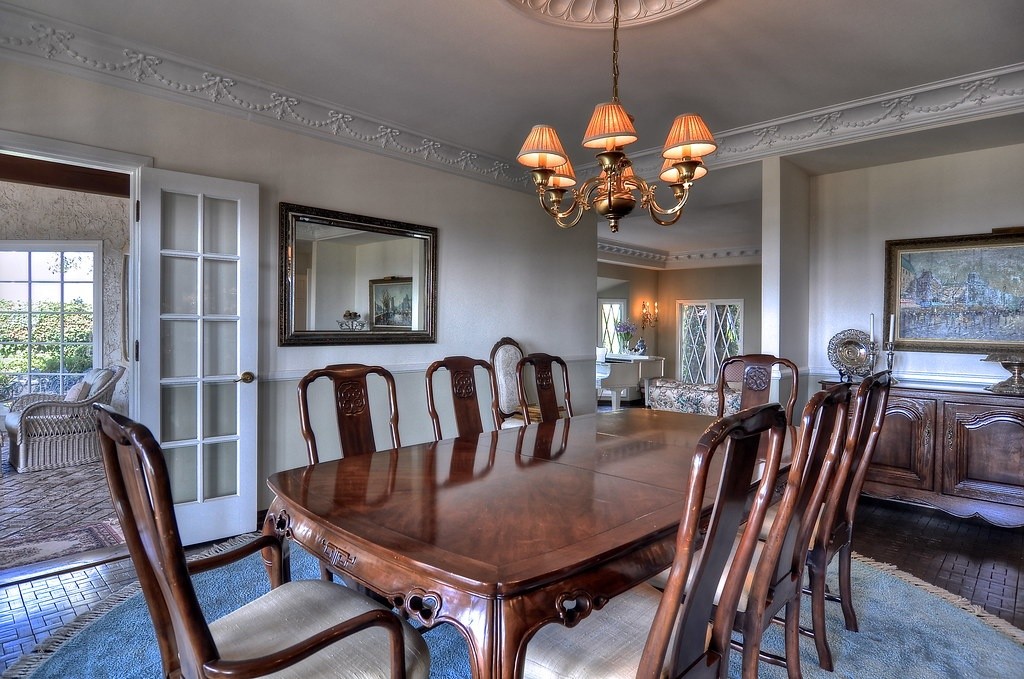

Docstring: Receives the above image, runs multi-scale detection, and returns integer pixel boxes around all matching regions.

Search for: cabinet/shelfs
[817,376,1024,528]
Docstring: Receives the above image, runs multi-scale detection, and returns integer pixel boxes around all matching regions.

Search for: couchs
[645,378,742,417]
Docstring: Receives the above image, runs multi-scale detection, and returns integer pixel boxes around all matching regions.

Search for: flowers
[615,317,638,340]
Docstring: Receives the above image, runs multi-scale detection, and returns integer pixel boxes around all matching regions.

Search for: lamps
[641,301,658,330]
[516,0,718,233]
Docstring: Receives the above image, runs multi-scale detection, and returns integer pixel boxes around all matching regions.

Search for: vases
[619,340,630,354]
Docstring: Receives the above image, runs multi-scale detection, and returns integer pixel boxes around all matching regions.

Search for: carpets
[0,522,125,571]
[89,519,126,548]
[0,530,1024,679]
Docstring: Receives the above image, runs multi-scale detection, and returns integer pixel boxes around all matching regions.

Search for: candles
[870,314,874,342]
[889,313,895,342]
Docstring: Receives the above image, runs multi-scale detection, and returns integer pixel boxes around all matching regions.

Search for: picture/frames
[368,277,413,331]
[882,234,1024,354]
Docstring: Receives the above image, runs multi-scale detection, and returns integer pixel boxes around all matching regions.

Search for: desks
[261,412,821,679]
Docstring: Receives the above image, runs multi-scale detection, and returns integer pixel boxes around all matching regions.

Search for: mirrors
[277,202,438,346]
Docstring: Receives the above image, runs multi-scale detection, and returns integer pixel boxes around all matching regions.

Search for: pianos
[596,346,667,410]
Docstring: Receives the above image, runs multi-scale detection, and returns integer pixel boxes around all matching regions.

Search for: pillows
[63,381,91,418]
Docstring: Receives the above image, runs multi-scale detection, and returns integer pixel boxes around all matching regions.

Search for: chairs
[89,337,891,679]
[5,364,126,473]
